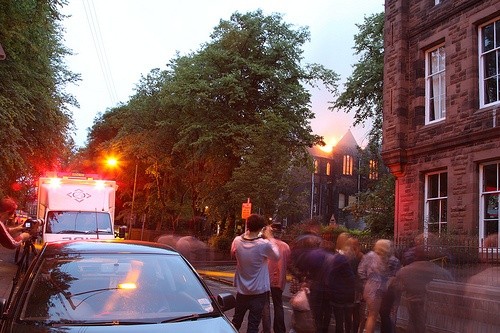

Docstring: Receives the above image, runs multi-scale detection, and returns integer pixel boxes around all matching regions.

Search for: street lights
[105,155,139,238]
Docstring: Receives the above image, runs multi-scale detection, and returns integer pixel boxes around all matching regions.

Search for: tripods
[0,232,39,333]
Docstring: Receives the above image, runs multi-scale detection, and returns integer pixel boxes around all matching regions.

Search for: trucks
[36,176,127,245]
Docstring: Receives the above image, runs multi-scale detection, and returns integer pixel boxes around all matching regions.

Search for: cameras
[21,218,45,232]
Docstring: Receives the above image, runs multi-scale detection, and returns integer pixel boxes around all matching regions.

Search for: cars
[1,238,241,333]
